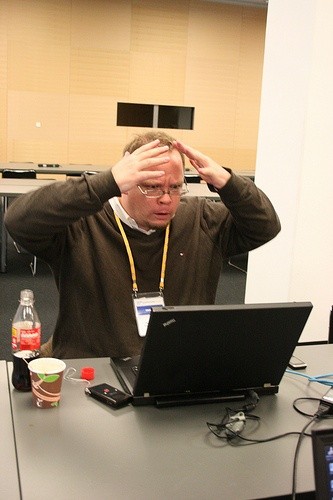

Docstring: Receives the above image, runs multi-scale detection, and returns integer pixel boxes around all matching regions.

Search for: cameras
[86,382,133,409]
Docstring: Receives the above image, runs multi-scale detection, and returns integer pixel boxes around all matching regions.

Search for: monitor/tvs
[116,102,154,127]
[158,105,194,130]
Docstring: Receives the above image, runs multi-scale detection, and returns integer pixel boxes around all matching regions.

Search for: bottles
[12,289,42,392]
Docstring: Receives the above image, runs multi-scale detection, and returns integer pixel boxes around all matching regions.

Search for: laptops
[311,428,333,500]
[110,302,313,409]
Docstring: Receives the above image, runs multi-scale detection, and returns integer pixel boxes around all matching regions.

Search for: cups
[28,357,66,408]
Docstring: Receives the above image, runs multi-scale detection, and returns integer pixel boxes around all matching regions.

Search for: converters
[319,385,333,415]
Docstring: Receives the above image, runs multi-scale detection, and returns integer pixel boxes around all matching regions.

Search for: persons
[3,132,282,359]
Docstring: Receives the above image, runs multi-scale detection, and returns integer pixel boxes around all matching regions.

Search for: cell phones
[288,354,307,370]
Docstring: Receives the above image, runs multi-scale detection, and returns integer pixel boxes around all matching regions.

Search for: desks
[0,344,333,499]
[0,163,255,202]
[0,177,220,273]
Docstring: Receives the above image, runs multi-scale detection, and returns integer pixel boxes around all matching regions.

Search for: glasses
[137,176,189,198]
